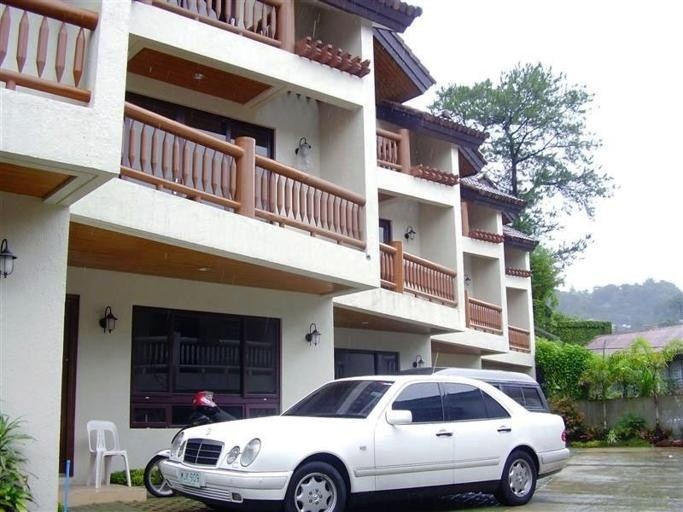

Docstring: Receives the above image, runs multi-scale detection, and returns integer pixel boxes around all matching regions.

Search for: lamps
[304,322,321,348]
[465,272,472,286]
[405,225,416,241]
[294,136,314,172]
[412,354,425,368]
[0,237,15,277]
[99,306,117,335]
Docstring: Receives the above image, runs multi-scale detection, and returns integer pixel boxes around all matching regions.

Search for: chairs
[85,419,132,490]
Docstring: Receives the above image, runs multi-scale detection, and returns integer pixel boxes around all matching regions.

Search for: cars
[158,374,571,512]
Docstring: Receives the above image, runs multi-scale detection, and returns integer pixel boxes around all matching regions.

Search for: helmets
[191,391,217,410]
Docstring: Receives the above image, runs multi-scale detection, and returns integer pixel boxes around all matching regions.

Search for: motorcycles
[144,391,236,497]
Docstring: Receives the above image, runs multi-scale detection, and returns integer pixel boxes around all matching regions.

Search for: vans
[401,366,550,413]
